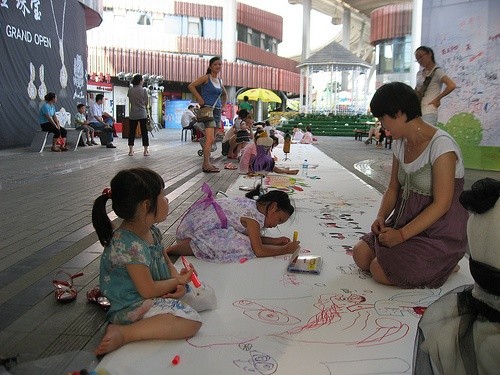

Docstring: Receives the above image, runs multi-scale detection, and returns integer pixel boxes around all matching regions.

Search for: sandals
[224,163,238,169]
[87,285,111,310]
[52,269,84,302]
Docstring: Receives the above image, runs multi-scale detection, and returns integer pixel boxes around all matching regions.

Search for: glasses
[415,52,428,62]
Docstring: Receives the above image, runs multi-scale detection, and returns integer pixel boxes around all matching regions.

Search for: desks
[362,122,375,131]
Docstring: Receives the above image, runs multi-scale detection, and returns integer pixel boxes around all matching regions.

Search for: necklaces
[407,127,430,152]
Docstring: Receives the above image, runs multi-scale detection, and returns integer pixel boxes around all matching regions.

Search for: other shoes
[91,140,98,145]
[61,147,68,151]
[104,126,115,132]
[86,141,93,146]
[365,139,371,144]
[107,144,116,148]
[52,147,60,152]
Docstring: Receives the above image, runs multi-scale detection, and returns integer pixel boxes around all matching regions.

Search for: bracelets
[399,228,406,243]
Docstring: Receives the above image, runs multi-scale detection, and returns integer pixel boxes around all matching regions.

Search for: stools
[355,130,369,141]
[182,128,190,141]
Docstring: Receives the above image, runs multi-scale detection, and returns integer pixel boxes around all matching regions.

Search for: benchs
[30,128,102,153]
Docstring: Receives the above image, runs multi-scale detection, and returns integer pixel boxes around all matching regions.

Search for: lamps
[116,72,165,94]
[138,11,151,25]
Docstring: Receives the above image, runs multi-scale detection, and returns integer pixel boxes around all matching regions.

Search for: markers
[181,255,202,288]
[292,230,298,242]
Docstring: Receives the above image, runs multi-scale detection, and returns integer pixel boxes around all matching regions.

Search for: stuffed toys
[412,179,500,375]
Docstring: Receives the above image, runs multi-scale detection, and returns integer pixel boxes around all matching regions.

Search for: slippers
[203,167,220,172]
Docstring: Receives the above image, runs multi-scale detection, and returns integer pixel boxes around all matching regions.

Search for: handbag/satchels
[146,116,156,131]
[56,134,64,145]
[196,104,214,122]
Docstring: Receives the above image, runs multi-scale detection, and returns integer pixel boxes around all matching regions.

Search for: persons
[364,121,386,147]
[220,96,317,175]
[127,74,150,156]
[167,183,301,262]
[74,93,118,148]
[282,134,291,154]
[352,82,469,289]
[92,168,215,354]
[415,46,456,126]
[181,104,229,142]
[39,93,68,152]
[188,56,223,173]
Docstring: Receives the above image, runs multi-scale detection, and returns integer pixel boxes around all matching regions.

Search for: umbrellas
[236,87,282,120]
